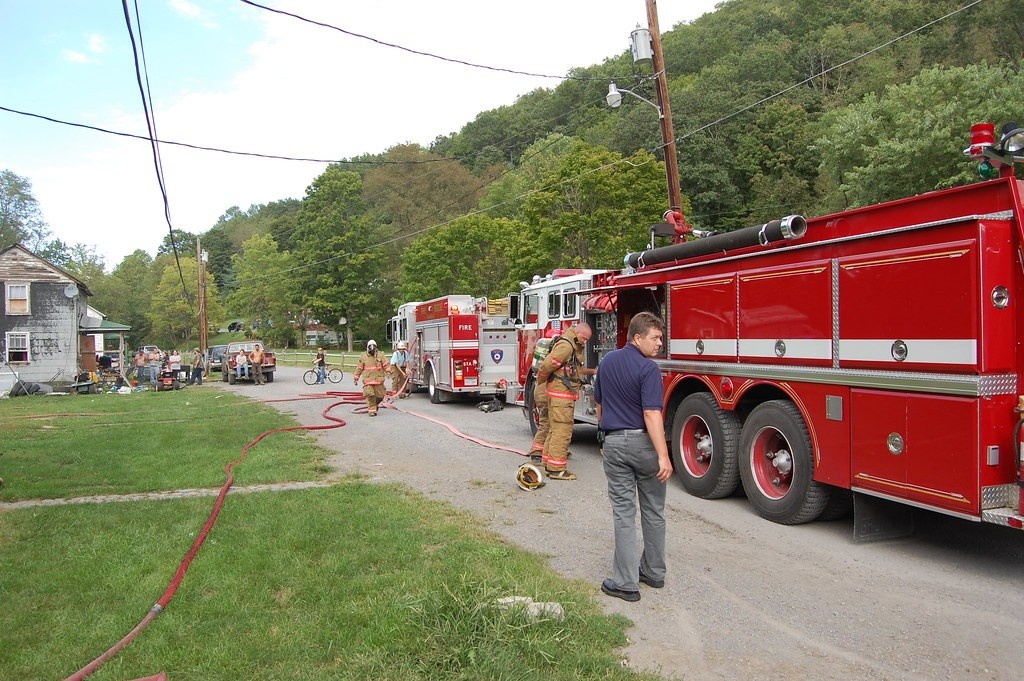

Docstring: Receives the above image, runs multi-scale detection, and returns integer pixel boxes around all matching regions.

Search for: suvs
[208,345,228,372]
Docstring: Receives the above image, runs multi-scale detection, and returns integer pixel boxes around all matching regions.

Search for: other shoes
[370,412,376,417]
[260,382,265,384]
[255,382,258,385]
[531,456,541,466]
[545,469,577,480]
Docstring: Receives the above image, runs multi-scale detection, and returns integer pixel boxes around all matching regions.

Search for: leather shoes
[603,579,642,601]
[638,567,665,588]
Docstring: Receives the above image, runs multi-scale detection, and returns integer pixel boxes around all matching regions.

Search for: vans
[135,345,158,357]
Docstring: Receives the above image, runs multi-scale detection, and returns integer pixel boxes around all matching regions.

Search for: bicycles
[303,361,343,385]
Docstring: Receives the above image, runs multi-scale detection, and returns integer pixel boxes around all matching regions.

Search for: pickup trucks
[218,341,276,384]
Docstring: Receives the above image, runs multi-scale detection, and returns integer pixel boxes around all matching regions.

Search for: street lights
[604,81,682,209]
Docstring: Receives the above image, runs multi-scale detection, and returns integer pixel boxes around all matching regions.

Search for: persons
[532,322,599,481]
[390,342,412,399]
[249,344,265,385]
[353,340,393,417]
[235,349,249,379]
[594,313,673,601]
[134,348,204,385]
[312,348,326,384]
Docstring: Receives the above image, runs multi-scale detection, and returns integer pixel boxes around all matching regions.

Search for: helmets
[515,463,543,491]
[368,340,377,347]
[397,343,406,350]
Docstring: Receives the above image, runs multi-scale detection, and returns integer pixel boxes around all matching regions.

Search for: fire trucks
[505,122,1024,541]
[384,294,521,404]
